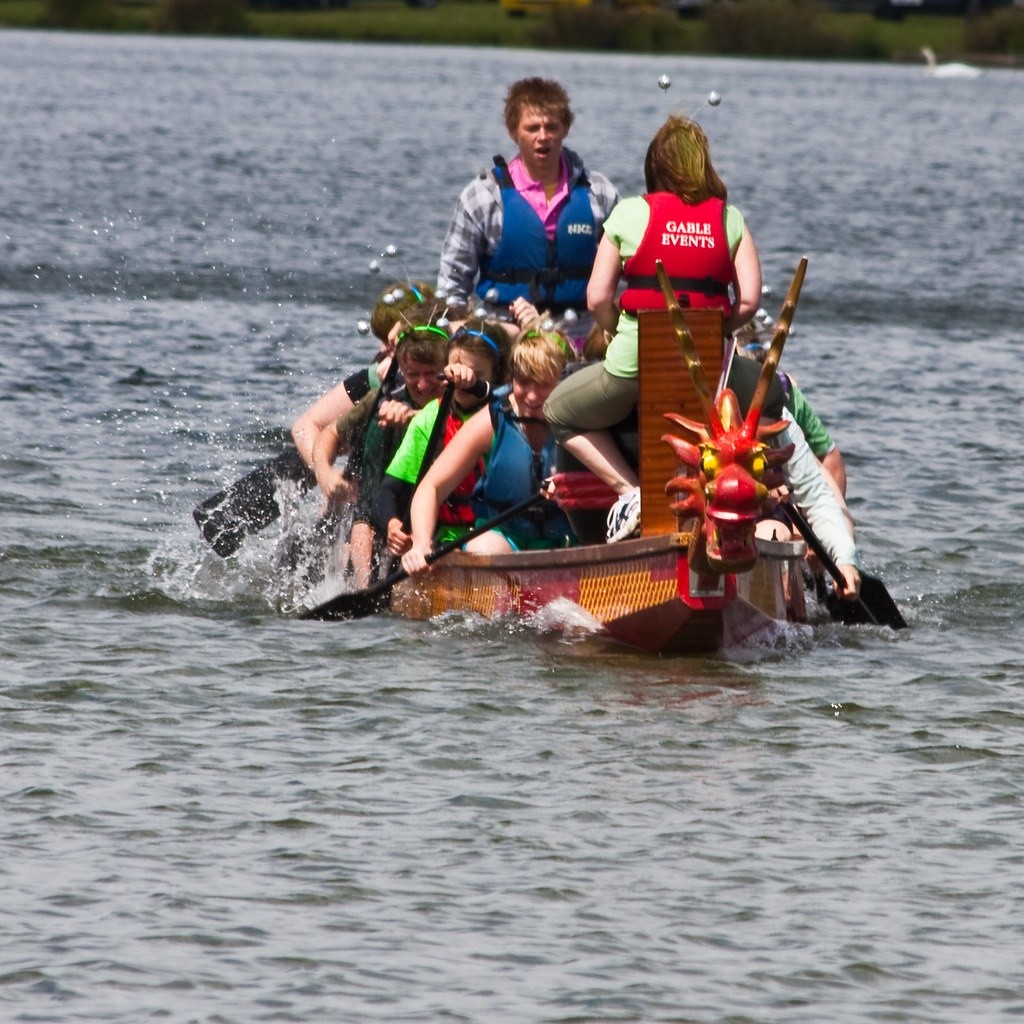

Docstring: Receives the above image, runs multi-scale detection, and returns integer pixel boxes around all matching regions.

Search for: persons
[287,72,862,602]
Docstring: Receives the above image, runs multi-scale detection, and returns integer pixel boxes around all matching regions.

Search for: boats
[386,253,812,663]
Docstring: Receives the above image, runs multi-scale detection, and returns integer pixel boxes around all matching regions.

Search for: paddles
[294,486,545,630]
[774,480,915,635]
[311,340,402,542]
[190,445,322,563]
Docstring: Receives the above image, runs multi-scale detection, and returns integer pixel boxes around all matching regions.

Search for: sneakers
[606,486,641,544]
